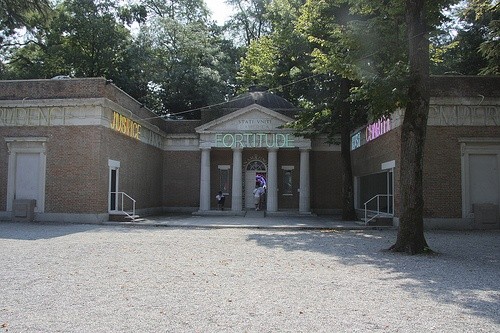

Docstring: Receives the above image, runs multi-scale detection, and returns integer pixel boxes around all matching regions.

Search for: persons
[253,182,265,211]
[216,192,225,211]
